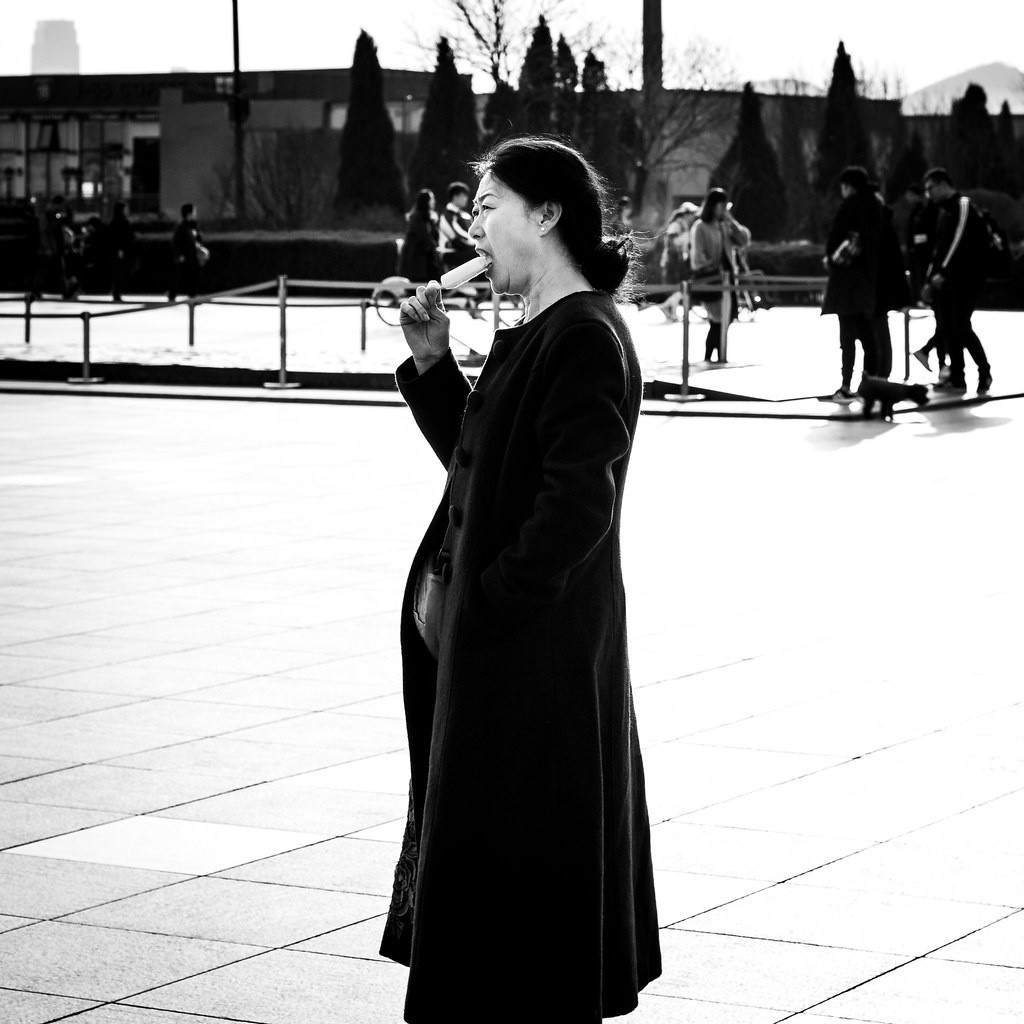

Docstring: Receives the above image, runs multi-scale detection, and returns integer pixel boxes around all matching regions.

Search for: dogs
[856,378,930,420]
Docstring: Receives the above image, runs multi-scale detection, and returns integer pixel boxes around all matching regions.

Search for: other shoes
[913,349,932,372]
[932,375,968,392]
[938,364,950,378]
[817,386,856,402]
[976,372,992,395]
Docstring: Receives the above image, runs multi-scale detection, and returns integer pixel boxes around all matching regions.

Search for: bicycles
[375,275,522,327]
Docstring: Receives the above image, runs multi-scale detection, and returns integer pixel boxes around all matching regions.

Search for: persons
[614,197,633,230]
[689,187,749,362]
[904,169,992,394]
[400,182,474,298]
[821,167,911,401]
[18,195,135,292]
[658,202,696,323]
[378,140,662,1024]
[165,204,203,300]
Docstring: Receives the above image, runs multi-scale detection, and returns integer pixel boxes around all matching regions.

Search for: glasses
[926,181,943,193]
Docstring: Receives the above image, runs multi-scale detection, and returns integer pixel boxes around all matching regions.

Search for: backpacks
[960,194,1015,281]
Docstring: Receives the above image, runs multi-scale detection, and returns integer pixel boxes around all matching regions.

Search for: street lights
[400,91,417,203]
[212,73,250,219]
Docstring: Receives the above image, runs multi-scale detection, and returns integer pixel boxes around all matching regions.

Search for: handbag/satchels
[196,241,210,263]
[687,264,723,306]
[824,231,860,270]
